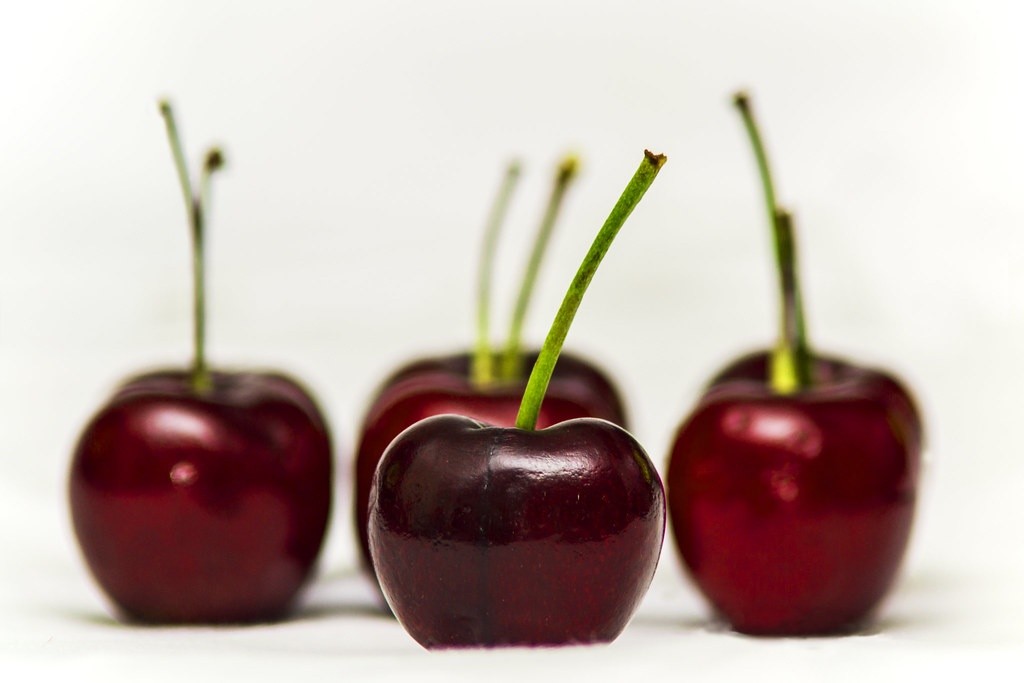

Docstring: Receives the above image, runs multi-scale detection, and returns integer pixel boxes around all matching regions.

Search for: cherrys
[71,99,922,648]
